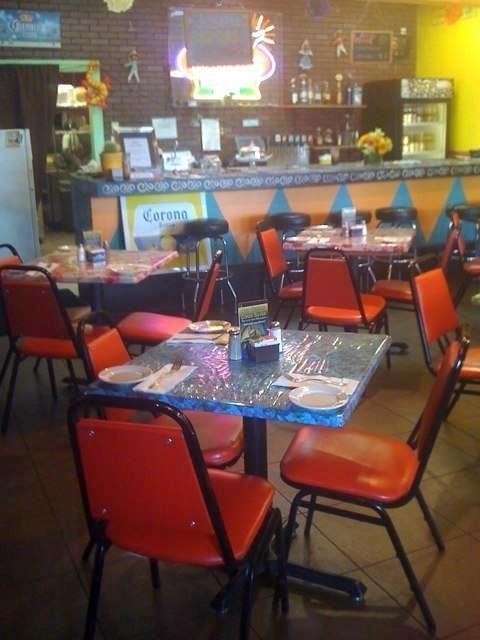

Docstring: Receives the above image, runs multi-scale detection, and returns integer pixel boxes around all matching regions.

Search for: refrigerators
[366,77,454,159]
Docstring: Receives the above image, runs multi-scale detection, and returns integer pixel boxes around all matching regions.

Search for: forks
[148,357,182,389]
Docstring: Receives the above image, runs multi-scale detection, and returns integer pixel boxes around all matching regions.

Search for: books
[374,228,414,243]
[165,326,239,344]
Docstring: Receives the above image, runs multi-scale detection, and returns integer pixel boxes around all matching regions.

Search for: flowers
[355,128,392,155]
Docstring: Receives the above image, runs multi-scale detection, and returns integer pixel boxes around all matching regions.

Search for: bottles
[78,244,86,262]
[351,128,360,145]
[102,240,112,258]
[322,81,332,105]
[289,77,299,105]
[324,127,334,143]
[307,78,313,104]
[335,73,343,104]
[345,86,352,106]
[361,219,368,235]
[352,82,363,106]
[229,329,243,361]
[402,132,436,154]
[271,133,312,166]
[403,107,440,122]
[270,320,283,352]
[343,221,350,237]
[315,126,323,146]
[298,73,309,104]
[313,80,321,103]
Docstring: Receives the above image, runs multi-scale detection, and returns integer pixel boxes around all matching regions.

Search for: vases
[364,152,383,167]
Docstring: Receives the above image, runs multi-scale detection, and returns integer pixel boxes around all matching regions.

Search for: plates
[187,319,231,333]
[97,364,154,386]
[288,384,348,409]
[285,236,311,244]
[107,262,151,275]
[382,235,413,243]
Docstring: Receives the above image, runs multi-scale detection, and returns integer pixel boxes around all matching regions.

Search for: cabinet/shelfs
[0,59,106,231]
[287,103,367,151]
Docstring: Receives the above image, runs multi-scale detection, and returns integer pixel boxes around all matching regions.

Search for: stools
[446,204,480,264]
[369,207,423,279]
[261,211,312,300]
[181,218,237,319]
[329,210,376,293]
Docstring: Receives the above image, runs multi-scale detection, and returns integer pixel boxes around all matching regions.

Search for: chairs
[256,220,325,331]
[450,209,480,306]
[78,312,243,559]
[65,399,296,640]
[1,243,80,393]
[114,251,223,357]
[370,223,461,334]
[272,331,468,634]
[298,248,391,369]
[406,254,480,451]
[0,265,110,439]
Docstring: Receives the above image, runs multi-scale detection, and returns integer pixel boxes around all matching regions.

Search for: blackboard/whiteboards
[183,8,253,68]
[349,29,394,66]
